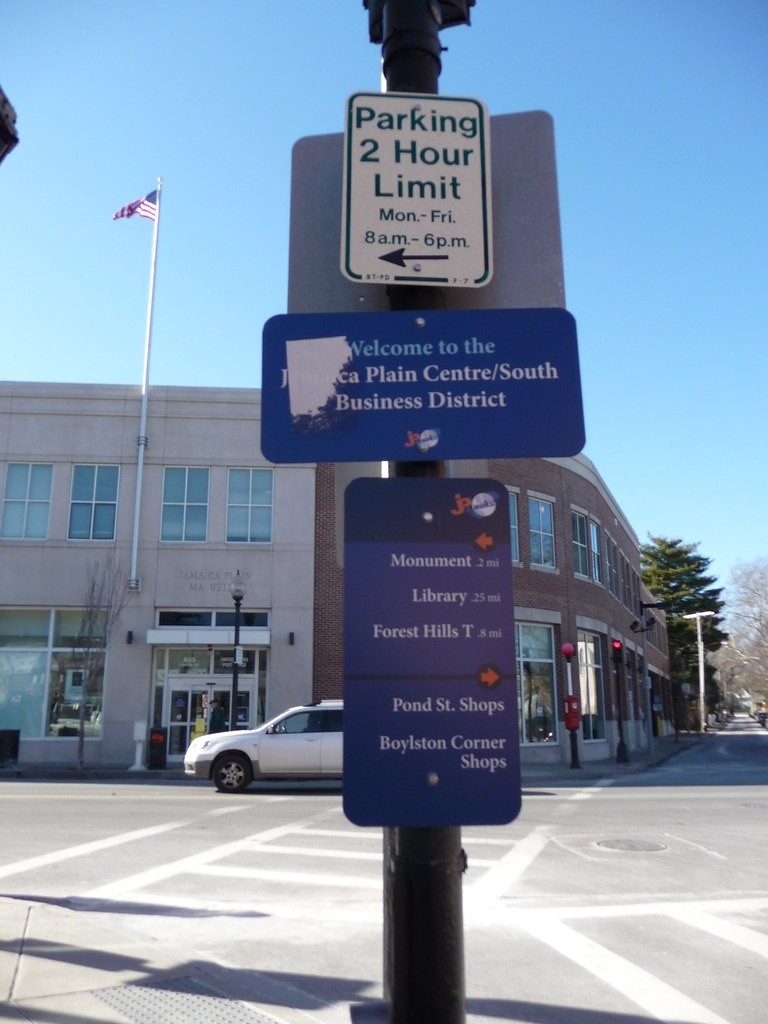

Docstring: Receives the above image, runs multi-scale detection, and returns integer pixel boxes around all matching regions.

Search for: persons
[208,700,225,734]
[303,713,319,732]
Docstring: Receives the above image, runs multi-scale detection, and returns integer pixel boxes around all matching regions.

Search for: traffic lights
[612,638,622,652]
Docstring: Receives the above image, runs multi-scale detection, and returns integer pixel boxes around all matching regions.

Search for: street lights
[225,568,247,731]
[630,598,681,758]
[721,655,762,700]
[682,608,715,732]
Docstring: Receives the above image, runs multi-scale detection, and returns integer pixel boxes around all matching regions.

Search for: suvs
[183,700,346,794]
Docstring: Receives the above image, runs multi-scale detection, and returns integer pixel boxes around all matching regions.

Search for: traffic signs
[336,91,495,290]
[336,474,524,826]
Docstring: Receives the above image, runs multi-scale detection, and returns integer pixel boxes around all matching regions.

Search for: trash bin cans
[149,727,168,770]
[0,728,21,769]
